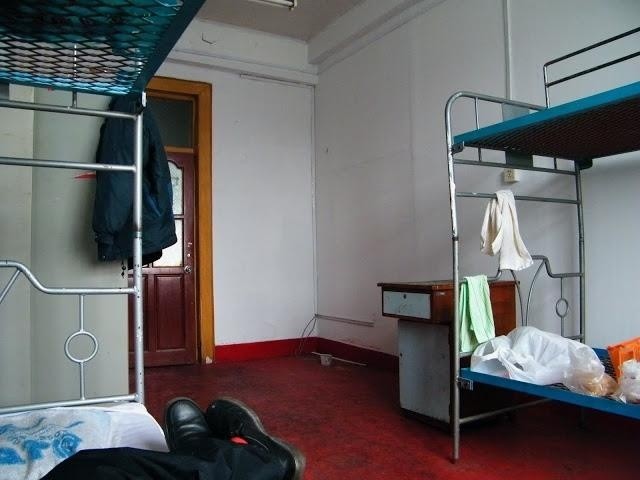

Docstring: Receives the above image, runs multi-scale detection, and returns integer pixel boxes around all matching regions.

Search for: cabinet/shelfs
[1,35,138,407]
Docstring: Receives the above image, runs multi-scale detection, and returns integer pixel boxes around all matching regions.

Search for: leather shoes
[163,396,211,452]
[205,397,304,476]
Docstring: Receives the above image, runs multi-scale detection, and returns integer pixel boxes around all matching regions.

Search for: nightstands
[376,277,520,429]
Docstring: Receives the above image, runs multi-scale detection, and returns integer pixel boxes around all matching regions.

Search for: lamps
[254,1,298,11]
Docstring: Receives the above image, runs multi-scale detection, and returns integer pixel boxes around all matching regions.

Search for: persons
[37,397,306,480]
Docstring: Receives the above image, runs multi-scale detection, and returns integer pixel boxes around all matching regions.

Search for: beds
[446,27,640,459]
[0,0,206,480]
